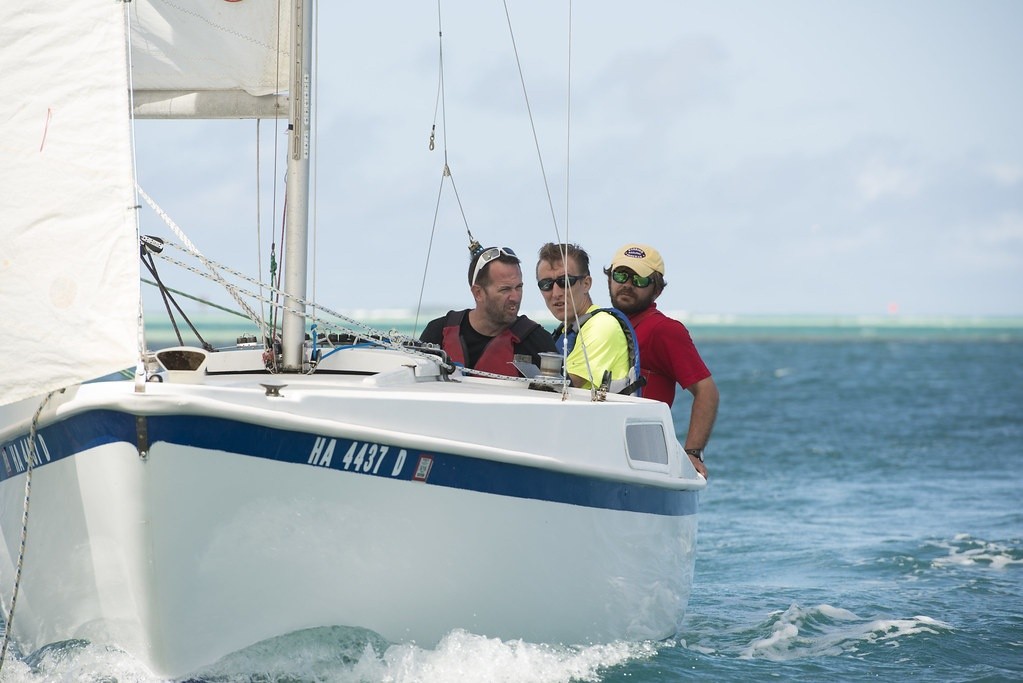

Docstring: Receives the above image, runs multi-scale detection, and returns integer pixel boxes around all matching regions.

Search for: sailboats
[0,0,706,682]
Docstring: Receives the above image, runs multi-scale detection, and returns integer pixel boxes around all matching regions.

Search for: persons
[415,246,573,386]
[535,242,641,397]
[602,242,719,480]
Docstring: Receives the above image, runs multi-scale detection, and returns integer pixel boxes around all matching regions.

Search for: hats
[612,243,665,279]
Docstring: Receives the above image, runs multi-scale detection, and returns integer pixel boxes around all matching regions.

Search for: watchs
[684,448,705,463]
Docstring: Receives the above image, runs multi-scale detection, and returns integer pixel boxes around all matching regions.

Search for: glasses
[471,247,519,290]
[537,271,588,292]
[611,269,656,288]
